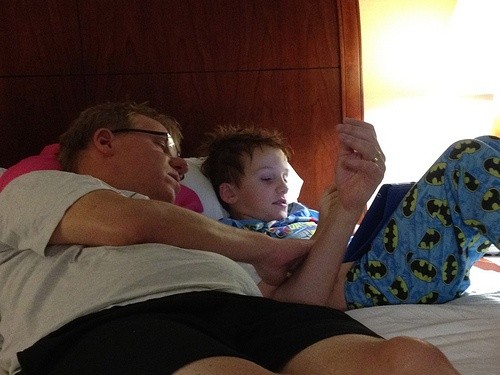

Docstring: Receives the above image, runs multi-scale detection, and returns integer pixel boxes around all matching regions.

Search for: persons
[205,128,500,309]
[1,103,463,375]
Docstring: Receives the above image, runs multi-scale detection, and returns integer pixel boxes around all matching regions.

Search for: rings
[372,149,382,161]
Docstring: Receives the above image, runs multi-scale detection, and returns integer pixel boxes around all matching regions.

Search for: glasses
[112,128,185,159]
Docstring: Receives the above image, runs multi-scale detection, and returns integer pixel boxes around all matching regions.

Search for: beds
[0,0,500,375]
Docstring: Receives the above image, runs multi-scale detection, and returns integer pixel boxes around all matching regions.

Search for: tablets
[342,183,417,263]
